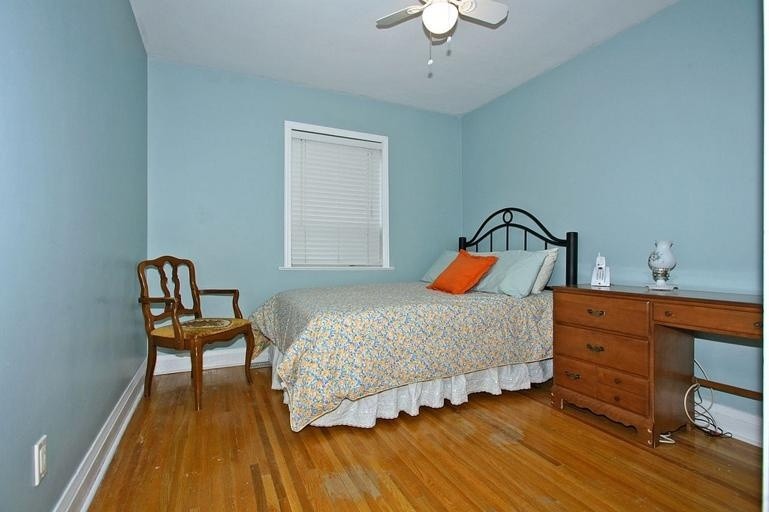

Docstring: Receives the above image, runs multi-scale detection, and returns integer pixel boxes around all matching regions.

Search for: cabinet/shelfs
[549,285,762,449]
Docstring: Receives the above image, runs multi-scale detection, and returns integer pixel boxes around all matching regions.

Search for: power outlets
[32,435,49,485]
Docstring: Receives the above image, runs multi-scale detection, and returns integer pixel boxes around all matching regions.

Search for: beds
[248,206,578,434]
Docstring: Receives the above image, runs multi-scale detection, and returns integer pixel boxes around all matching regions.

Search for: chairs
[137,255,255,411]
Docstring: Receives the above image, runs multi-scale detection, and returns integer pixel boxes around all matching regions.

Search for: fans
[375,1,509,30]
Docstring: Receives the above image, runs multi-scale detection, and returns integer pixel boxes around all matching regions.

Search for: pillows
[426,249,499,295]
[530,247,558,294]
[418,249,549,297]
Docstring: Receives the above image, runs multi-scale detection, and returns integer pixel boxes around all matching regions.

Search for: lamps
[641,240,680,290]
[422,1,460,66]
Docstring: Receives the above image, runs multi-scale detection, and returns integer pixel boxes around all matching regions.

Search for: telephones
[590,253,612,288]
